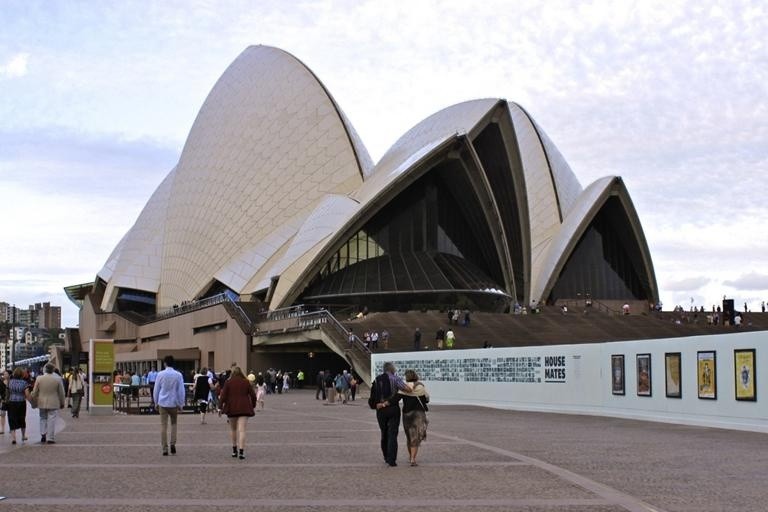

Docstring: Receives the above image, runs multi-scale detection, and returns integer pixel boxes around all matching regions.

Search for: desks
[111,381,198,416]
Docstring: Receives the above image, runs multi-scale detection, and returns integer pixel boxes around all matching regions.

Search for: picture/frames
[664,352,683,398]
[697,350,716,399]
[611,354,625,395]
[733,348,757,402]
[635,353,652,397]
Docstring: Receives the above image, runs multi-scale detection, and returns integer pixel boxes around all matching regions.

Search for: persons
[113,367,159,400]
[561,305,567,314]
[297,369,304,390]
[248,370,267,409]
[648,301,766,327]
[153,356,185,456]
[265,368,290,394]
[368,362,430,468]
[435,327,455,348]
[1,363,87,446]
[217,367,257,461]
[413,328,421,352]
[173,298,196,315]
[623,302,630,315]
[348,327,389,352]
[193,362,237,422]
[315,370,356,402]
[448,309,471,327]
[514,299,543,314]
